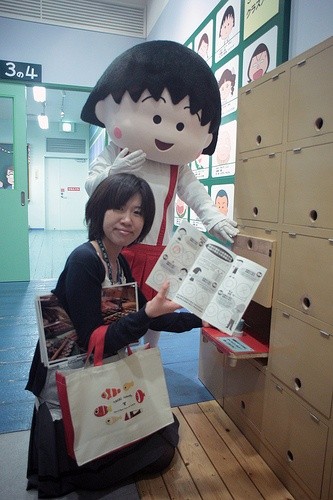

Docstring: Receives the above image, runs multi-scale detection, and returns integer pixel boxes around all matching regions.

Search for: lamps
[37,102,49,131]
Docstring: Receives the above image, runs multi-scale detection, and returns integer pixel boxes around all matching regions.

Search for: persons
[24,175,216,500]
[80,41,240,350]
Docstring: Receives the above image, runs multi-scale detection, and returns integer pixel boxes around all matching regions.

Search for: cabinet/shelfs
[198,35,332,500]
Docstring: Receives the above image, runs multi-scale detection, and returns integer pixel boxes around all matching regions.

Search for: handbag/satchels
[55,326,174,467]
